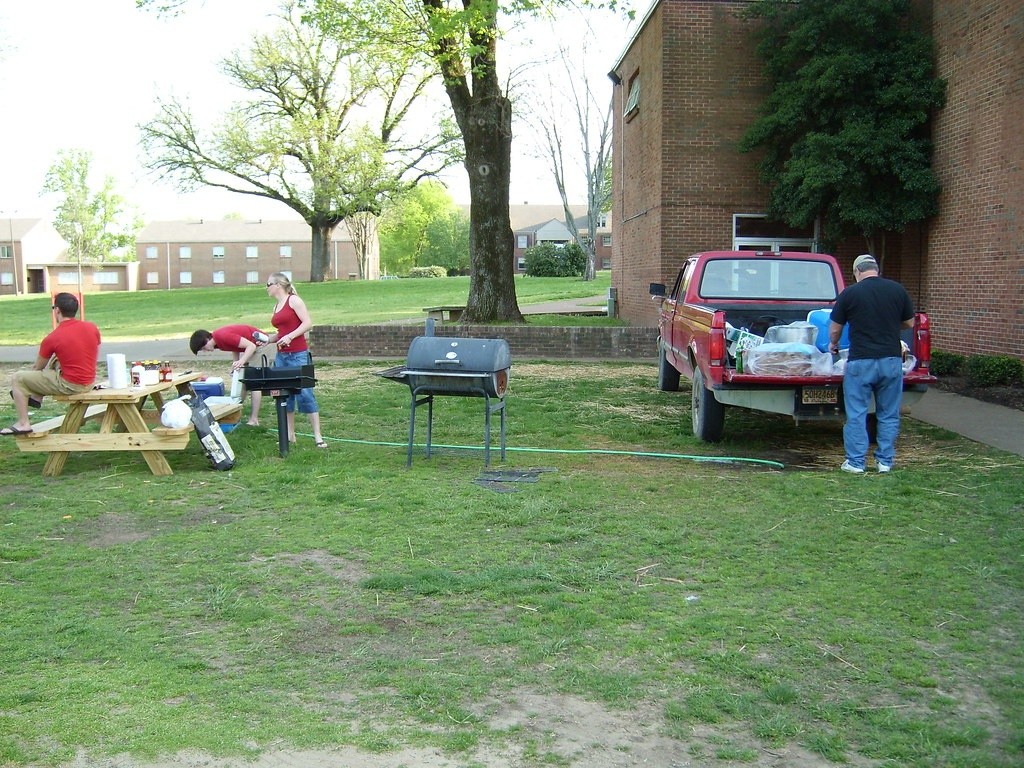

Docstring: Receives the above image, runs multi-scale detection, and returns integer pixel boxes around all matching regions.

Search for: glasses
[267,283,276,287]
[51,305,56,309]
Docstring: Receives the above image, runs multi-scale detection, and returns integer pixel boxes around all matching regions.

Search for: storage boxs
[807,309,851,353]
[189,381,225,399]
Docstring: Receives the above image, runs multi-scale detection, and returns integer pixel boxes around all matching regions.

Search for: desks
[41,370,203,476]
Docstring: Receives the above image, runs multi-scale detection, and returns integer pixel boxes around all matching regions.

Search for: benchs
[423,305,466,325]
[15,404,244,451]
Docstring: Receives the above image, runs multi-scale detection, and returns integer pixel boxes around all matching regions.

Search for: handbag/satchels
[161,394,192,428]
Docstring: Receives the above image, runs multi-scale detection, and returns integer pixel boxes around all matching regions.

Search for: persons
[828,254,915,474]
[256,272,327,448]
[189,323,277,426]
[0,292,102,435]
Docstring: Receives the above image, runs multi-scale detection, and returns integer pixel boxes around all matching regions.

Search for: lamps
[608,70,622,87]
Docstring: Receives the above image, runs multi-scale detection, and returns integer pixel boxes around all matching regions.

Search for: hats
[853,254,876,272]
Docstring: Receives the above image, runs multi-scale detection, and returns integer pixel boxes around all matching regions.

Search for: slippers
[276,440,295,445]
[10,390,41,408]
[0,426,33,435]
[316,442,327,448]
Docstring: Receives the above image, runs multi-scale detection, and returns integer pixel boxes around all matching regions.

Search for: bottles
[131,360,172,387]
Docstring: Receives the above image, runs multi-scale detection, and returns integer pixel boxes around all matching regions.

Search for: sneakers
[841,459,865,474]
[876,462,890,473]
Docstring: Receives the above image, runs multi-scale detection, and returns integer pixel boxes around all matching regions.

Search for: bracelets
[285,333,293,341]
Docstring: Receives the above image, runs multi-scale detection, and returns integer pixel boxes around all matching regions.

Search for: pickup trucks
[647,250,939,444]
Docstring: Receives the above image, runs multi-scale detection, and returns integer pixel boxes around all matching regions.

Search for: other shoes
[246,422,259,427]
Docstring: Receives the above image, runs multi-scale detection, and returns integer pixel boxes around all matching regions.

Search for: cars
[379,276,401,279]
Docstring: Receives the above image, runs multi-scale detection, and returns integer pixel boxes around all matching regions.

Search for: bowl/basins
[768,325,818,347]
